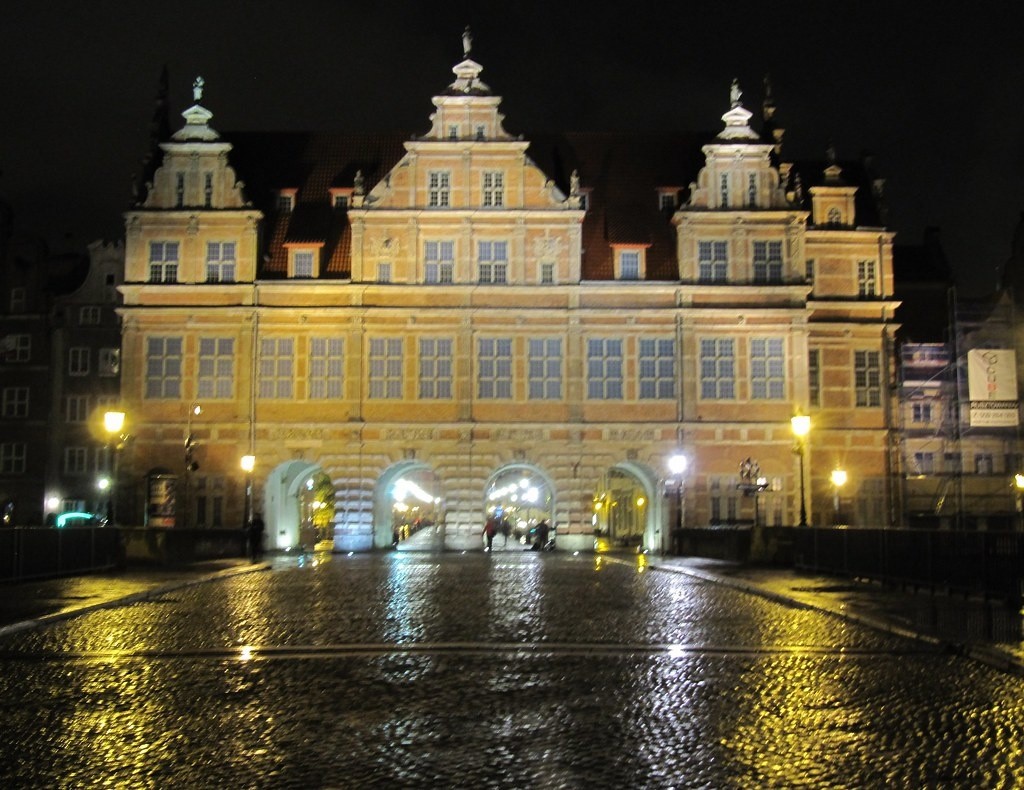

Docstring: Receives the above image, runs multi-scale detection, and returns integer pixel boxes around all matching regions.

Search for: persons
[481,516,497,554]
[501,518,512,547]
[247,513,265,564]
[534,520,549,551]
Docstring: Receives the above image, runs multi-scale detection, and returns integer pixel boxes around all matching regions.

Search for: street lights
[241,452,257,524]
[105,410,130,525]
[788,413,809,528]
